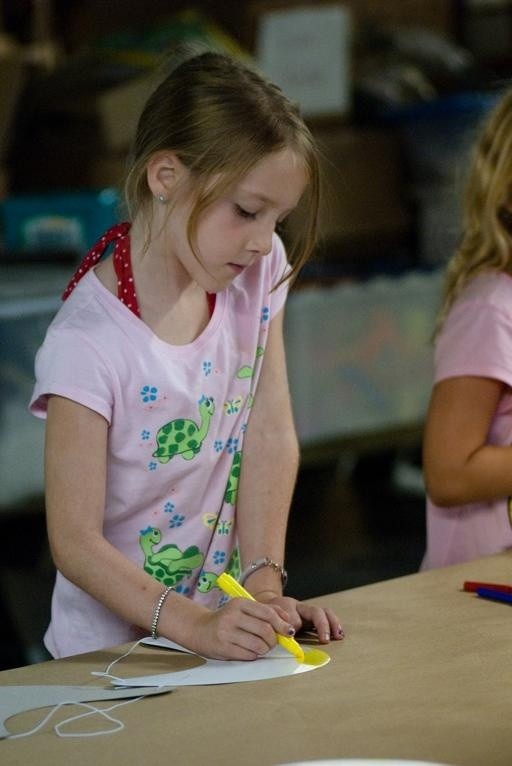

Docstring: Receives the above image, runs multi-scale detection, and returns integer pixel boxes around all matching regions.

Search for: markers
[216,572,304,657]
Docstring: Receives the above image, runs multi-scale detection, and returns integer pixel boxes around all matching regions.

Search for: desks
[0,548,511,766]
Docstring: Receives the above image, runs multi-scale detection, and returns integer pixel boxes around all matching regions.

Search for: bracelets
[237,557,288,587]
[152,586,176,639]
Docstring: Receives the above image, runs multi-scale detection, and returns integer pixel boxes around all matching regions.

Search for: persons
[28,52,344,661]
[419,86,511,571]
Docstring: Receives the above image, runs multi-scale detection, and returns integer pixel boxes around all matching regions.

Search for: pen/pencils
[464,581,512,605]
